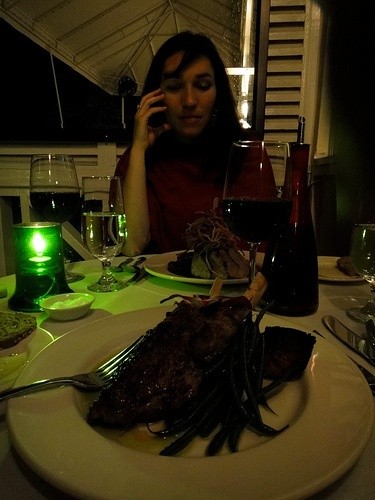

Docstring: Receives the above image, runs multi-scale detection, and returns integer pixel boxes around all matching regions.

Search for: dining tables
[0,255,375,500]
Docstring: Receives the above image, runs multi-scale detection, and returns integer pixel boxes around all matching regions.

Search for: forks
[0,333,145,402]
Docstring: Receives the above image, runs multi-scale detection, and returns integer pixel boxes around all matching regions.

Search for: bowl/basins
[41,293,96,321]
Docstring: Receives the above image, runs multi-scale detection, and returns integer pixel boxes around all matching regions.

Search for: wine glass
[346,224,375,323]
[82,176,130,293]
[29,153,85,284]
[221,139,294,311]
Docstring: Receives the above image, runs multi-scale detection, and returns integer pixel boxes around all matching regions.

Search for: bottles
[256,141,320,317]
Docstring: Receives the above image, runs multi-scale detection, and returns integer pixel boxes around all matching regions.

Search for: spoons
[110,256,146,273]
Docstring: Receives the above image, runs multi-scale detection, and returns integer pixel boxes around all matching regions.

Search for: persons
[109,30,279,259]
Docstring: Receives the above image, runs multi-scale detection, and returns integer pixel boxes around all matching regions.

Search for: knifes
[322,312,375,367]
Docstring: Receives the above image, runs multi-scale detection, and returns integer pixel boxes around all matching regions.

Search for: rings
[136,105,142,109]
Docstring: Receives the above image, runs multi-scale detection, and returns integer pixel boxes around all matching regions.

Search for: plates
[143,249,265,285]
[0,326,54,392]
[5,304,375,500]
[317,255,365,281]
